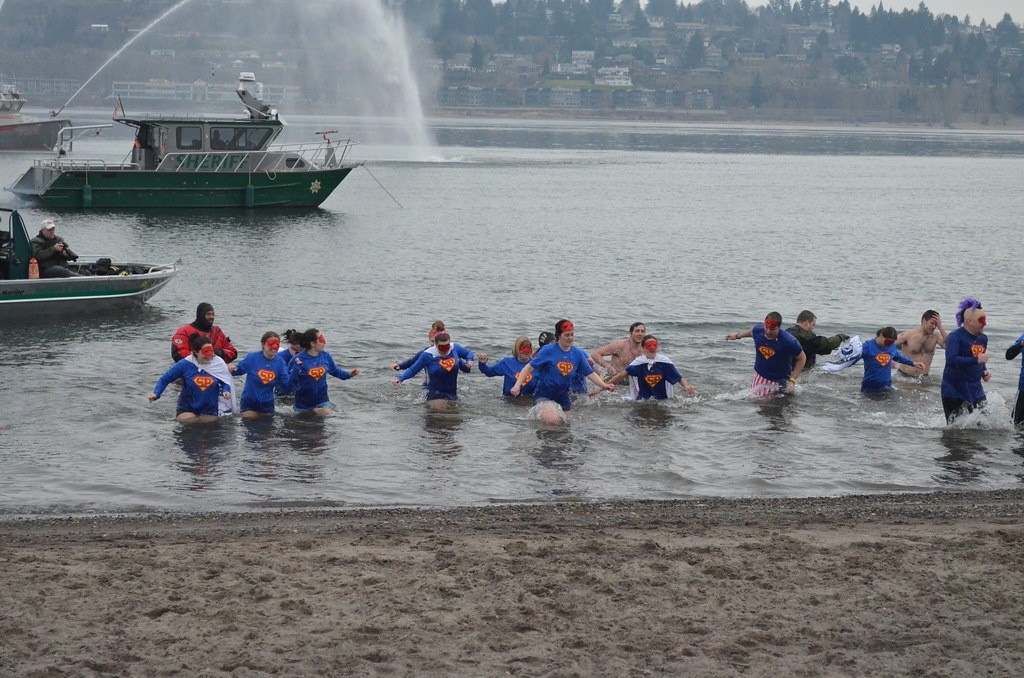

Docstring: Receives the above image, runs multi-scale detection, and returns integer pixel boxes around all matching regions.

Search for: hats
[538,331,557,346]
[41,219,55,231]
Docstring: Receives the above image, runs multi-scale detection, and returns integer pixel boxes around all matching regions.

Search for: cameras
[61,241,79,262]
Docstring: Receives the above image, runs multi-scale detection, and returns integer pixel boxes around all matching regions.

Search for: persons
[228,331,302,417]
[784,309,850,371]
[824,327,925,394]
[589,334,696,400]
[273,328,306,399]
[510,320,614,423]
[941,298,992,426]
[477,335,540,397]
[894,310,950,378]
[148,332,236,423]
[288,328,360,415]
[390,320,474,408]
[725,311,806,398]
[1005,332,1024,426]
[172,303,238,366]
[29,219,84,278]
[591,322,646,386]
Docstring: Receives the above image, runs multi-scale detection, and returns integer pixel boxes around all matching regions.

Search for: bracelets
[788,378,797,384]
[735,332,741,339]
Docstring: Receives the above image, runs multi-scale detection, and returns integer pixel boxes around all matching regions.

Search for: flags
[112,95,121,119]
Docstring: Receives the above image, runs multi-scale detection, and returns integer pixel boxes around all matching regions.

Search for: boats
[0,84,71,153]
[3,71,368,212]
[0,205,183,319]
